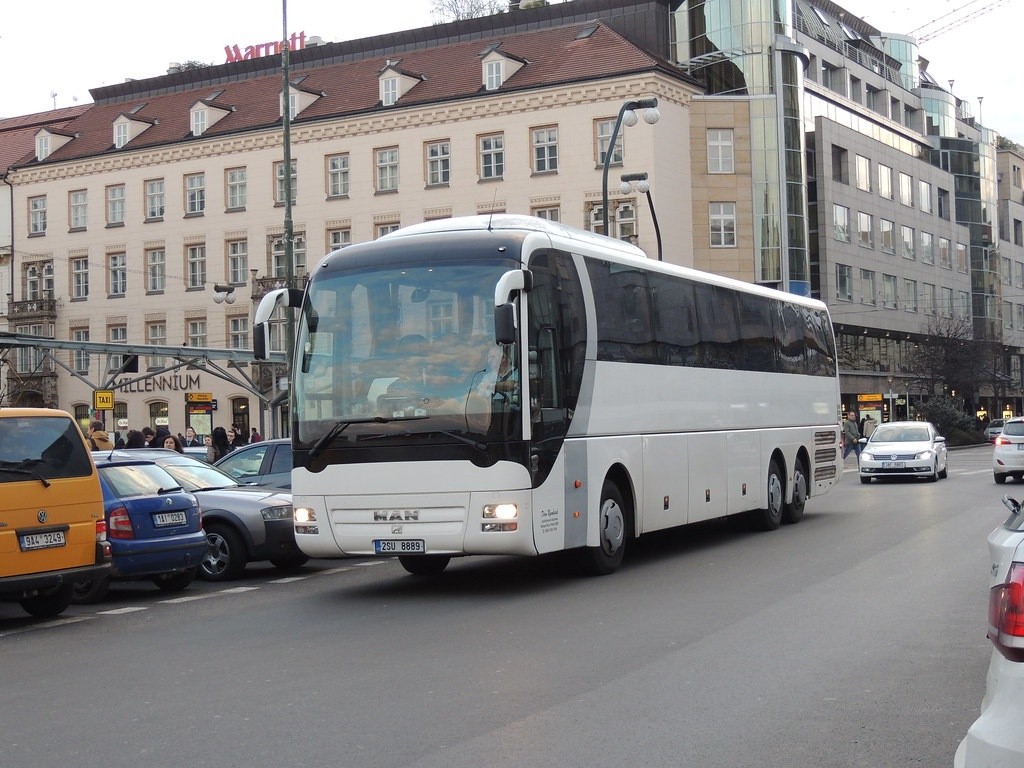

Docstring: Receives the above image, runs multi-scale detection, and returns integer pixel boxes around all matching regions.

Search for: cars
[992,416,1024,484]
[858,422,947,482]
[954,493,1024,768]
[68,453,209,606]
[212,437,292,491]
[983,418,1006,440]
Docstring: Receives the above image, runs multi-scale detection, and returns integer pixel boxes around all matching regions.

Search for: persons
[474,341,521,405]
[83,416,265,476]
[983,413,990,424]
[841,409,878,467]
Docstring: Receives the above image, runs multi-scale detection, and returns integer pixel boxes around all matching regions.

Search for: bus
[252,213,844,576]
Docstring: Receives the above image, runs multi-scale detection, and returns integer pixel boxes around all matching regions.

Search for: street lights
[602,96,661,235]
[621,171,662,260]
[887,376,969,422]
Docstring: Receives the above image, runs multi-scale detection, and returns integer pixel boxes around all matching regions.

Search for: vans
[1,405,114,618]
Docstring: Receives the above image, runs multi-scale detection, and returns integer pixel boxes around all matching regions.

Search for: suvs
[90,446,310,582]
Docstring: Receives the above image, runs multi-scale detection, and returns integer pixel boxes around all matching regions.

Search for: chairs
[397,334,431,357]
[466,334,496,357]
[525,349,541,407]
[369,378,400,412]
[432,332,467,356]
